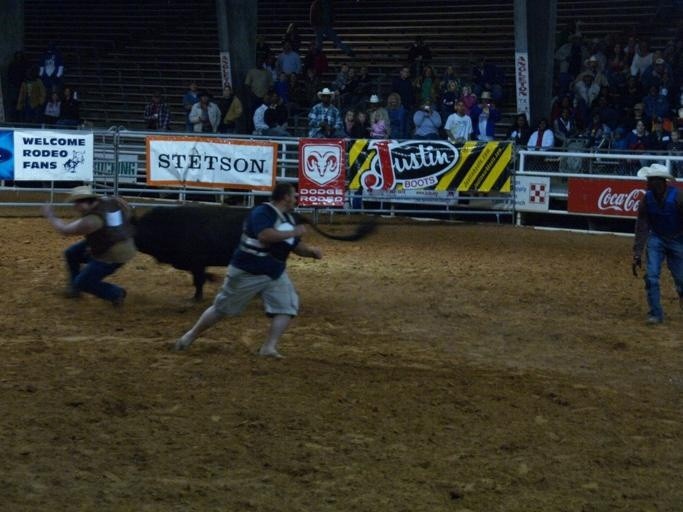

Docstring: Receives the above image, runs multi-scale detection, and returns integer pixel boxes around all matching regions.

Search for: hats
[318,88,336,99]
[637,163,674,181]
[366,94,384,103]
[581,56,601,77]
[63,186,96,202]
[480,91,493,100]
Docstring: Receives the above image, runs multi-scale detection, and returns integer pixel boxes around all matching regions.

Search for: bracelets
[632,254,641,260]
[307,246,315,258]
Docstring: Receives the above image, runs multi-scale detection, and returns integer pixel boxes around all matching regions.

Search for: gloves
[631,255,642,277]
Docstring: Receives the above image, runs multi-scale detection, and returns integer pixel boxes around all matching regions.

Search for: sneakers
[644,312,665,325]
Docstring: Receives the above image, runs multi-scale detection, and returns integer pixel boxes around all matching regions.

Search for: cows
[128,202,383,303]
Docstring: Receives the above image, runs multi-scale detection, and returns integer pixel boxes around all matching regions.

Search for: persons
[14,38,80,132]
[141,89,172,134]
[171,183,322,358]
[308,0,356,58]
[181,20,529,145]
[631,164,682,324]
[39,185,136,310]
[524,19,681,179]
